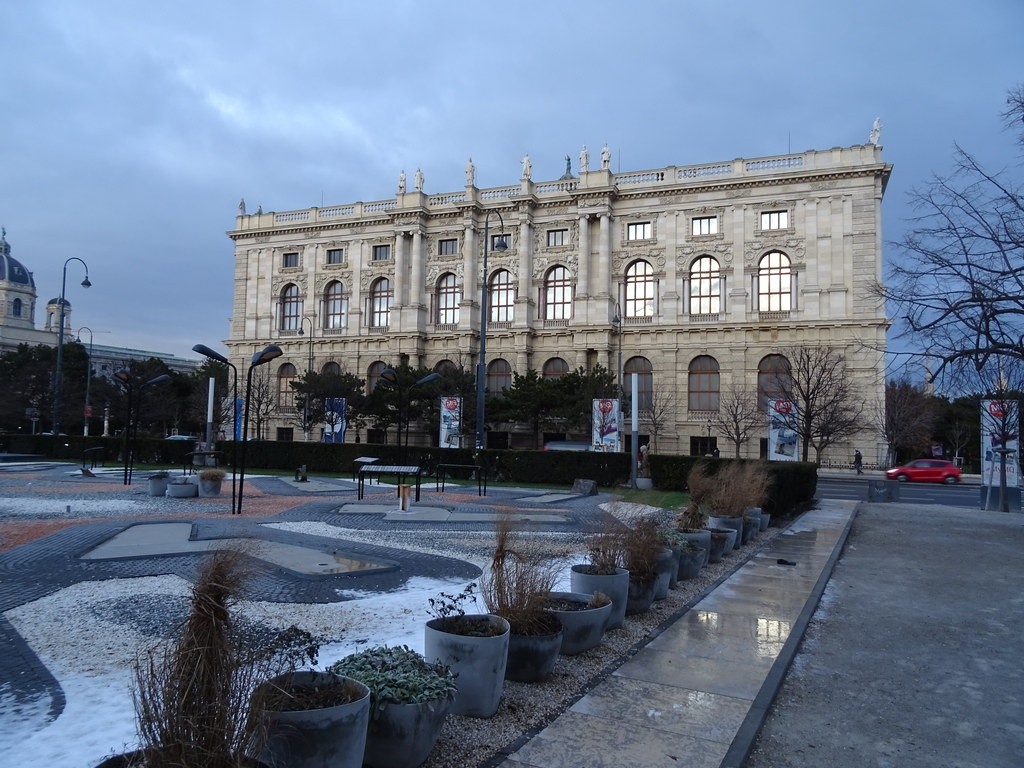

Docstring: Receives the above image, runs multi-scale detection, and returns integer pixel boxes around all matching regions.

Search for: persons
[852,448,865,476]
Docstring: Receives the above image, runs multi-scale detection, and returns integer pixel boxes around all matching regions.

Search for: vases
[709,534,728,563]
[712,527,737,555]
[636,477,653,489]
[742,516,760,545]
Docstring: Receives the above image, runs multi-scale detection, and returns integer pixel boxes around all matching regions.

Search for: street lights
[297,315,313,442]
[113,368,172,485]
[191,343,284,515]
[52,256,93,436]
[706,418,712,457]
[75,326,96,439]
[612,302,625,453]
[378,366,443,498]
[471,206,508,481]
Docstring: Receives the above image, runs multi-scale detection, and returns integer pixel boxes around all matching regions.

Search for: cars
[885,458,962,485]
[164,435,199,441]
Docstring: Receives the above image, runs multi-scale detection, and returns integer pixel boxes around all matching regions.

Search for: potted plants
[147,470,168,497]
[167,477,198,498]
[93,532,267,768]
[197,468,226,498]
[662,527,692,589]
[570,487,630,631]
[674,478,714,568]
[424,582,509,718]
[678,538,706,580]
[706,459,750,550]
[325,644,461,768]
[649,524,673,600]
[478,501,578,683]
[250,626,370,768]
[621,516,660,615]
[744,457,776,518]
[757,496,774,531]
[529,589,614,656]
